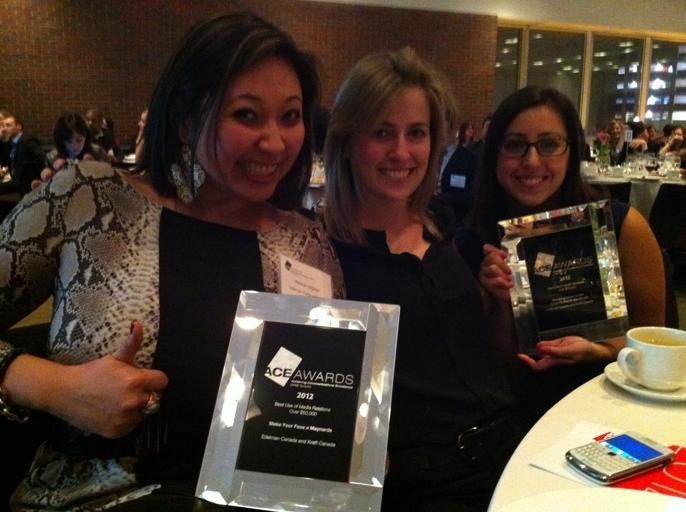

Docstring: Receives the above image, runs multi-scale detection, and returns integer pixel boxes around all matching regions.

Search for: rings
[142,387,162,417]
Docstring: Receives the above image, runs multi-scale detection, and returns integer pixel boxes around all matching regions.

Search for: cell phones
[564,430,676,487]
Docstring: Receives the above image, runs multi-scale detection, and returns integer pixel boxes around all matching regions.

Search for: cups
[622,326,686,392]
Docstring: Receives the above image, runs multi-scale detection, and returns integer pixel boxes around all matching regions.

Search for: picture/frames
[497,199,629,355]
[193,290,400,512]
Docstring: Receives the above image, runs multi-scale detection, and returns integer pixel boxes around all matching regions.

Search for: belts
[404,412,520,475]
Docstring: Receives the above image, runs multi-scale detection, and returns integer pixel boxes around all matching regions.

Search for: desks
[487,348,685,512]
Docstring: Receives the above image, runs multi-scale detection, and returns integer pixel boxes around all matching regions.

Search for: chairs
[648,184,685,273]
[587,181,631,204]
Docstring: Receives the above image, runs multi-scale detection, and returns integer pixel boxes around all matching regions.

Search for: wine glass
[585,153,680,178]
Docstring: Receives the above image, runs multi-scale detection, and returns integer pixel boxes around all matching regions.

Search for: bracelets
[597,338,619,359]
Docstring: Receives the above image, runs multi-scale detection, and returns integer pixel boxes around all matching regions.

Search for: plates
[605,360,686,404]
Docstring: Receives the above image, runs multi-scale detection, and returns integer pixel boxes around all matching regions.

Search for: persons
[1,110,685,195]
[322,43,525,512]
[0,10,349,510]
[479,85,668,377]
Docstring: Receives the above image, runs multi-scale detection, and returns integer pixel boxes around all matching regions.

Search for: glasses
[496,138,574,159]
